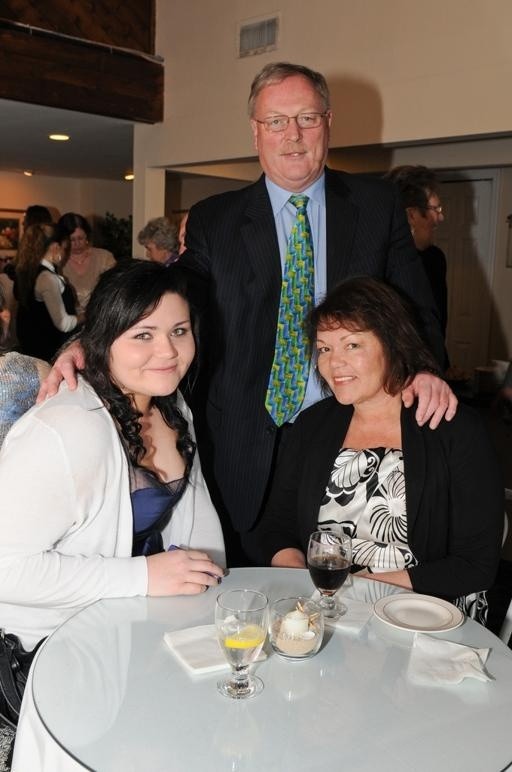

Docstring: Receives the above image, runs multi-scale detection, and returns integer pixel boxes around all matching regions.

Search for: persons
[0,203,188,361]
[252,275,498,631]
[36,62,460,567]
[380,164,448,353]
[0,256,228,732]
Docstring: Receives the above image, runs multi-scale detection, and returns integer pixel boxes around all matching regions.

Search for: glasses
[252,107,334,133]
[70,235,85,242]
[426,201,444,219]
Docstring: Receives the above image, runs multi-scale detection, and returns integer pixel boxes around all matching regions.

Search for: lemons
[223,622,266,649]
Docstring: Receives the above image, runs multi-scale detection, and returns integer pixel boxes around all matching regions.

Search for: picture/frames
[0,208,27,260]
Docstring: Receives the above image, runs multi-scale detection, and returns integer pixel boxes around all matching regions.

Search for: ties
[265,195,316,429]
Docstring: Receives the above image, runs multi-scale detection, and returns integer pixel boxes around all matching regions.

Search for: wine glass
[210,588,271,702]
[308,530,354,618]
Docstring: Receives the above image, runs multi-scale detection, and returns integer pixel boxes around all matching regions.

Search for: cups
[268,594,325,662]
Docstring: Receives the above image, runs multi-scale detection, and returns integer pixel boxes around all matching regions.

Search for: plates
[374,594,467,636]
